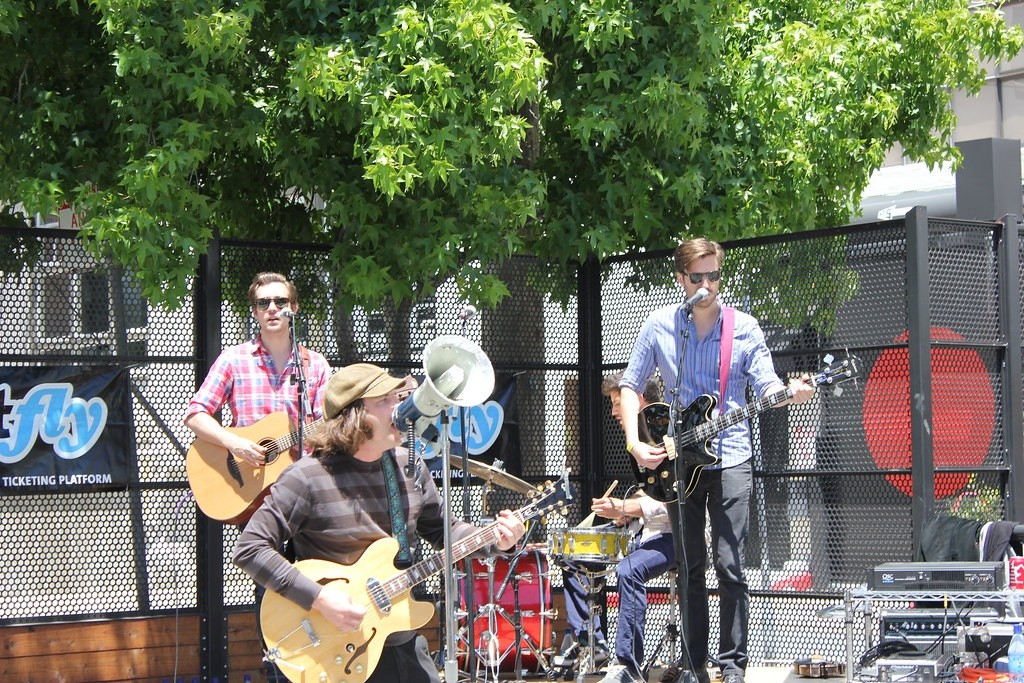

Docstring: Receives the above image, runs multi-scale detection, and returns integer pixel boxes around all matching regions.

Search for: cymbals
[447,451,540,498]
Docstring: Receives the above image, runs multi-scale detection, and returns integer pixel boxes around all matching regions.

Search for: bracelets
[626,440,638,452]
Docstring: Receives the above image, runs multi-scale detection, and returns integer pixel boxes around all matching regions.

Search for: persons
[180,274,334,683]
[617,237,819,683]
[552,370,678,683]
[232,364,525,683]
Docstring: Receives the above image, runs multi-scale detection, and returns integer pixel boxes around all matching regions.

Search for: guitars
[185,372,421,523]
[630,347,866,505]
[259,479,577,683]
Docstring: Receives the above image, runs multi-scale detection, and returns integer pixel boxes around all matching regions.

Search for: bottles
[560,628,574,656]
[1007,624,1024,683]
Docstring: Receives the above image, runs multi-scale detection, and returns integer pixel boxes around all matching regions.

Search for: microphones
[679,288,709,311]
[280,307,294,319]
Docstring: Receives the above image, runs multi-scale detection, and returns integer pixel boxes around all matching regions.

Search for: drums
[438,542,552,674]
[548,525,636,564]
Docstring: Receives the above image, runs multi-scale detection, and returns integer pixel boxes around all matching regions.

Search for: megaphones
[391,335,496,434]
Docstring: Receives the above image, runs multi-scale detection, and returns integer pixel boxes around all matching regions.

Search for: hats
[324,363,406,419]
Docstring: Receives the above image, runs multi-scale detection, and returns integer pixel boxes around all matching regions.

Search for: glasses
[682,268,721,284]
[254,297,290,310]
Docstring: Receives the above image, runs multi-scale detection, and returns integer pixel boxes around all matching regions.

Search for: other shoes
[723,674,745,683]
[678,669,710,683]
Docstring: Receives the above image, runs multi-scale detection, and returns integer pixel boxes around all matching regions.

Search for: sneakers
[594,662,644,683]
[554,640,609,673]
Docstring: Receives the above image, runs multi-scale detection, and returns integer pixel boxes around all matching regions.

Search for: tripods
[434,556,614,683]
